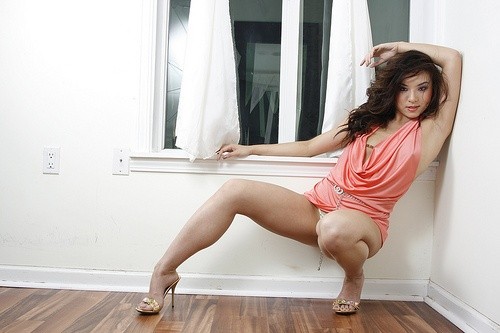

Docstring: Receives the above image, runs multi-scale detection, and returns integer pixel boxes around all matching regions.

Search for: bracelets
[396,42,399,54]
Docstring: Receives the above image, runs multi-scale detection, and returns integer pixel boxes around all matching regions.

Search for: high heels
[136,276,181,314]
[330,298,360,314]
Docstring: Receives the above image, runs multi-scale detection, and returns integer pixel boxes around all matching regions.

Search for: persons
[136,41,462,314]
